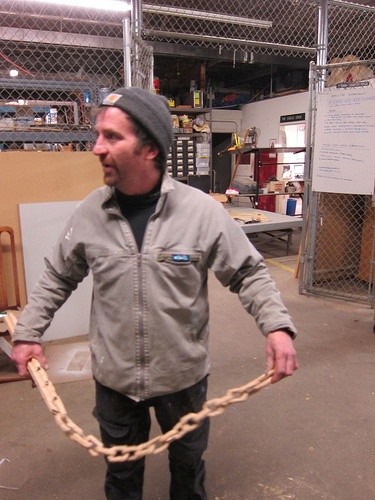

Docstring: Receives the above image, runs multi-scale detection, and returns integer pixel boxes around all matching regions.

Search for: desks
[224,207,303,255]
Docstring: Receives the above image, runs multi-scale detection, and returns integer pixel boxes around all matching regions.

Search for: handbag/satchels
[258,181,303,216]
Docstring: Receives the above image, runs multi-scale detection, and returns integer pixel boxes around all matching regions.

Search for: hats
[100,87,174,159]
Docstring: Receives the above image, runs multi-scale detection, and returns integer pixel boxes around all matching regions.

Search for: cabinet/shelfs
[166,108,212,182]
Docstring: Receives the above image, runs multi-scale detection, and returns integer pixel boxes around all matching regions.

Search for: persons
[11,88,298,500]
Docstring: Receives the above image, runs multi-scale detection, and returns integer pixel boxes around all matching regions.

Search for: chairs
[0,226,36,388]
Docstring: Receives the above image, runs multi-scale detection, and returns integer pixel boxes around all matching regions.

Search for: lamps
[142,3,272,29]
